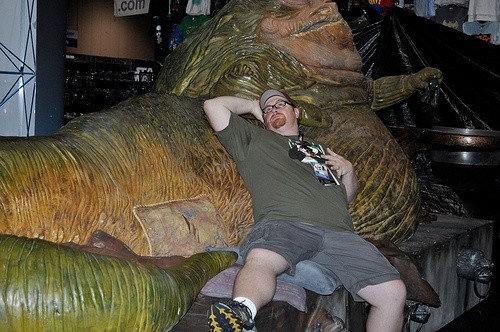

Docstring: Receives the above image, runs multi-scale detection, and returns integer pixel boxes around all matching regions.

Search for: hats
[259,89,294,110]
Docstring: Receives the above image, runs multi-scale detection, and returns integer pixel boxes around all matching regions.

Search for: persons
[203,88,406,332]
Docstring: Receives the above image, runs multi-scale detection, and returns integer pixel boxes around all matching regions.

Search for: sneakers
[207,299,258,332]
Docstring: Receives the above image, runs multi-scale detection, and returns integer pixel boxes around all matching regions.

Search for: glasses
[262,100,295,114]
